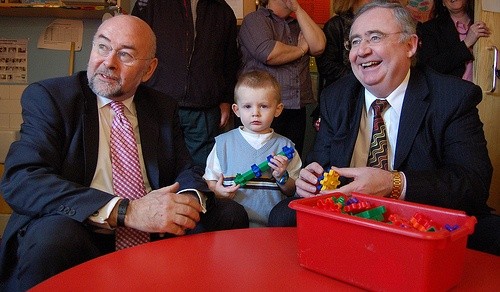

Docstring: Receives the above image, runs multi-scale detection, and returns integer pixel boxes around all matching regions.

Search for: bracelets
[390,171,402,199]
[299,46,306,58]
[117,198,129,228]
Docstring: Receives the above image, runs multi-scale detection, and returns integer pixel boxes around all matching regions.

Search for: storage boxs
[288,191,477,290]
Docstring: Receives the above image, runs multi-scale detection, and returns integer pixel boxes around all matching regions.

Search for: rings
[479,26,481,29]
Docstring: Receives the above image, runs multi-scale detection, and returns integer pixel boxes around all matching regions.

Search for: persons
[131,0,492,169]
[202,70,303,227]
[295,3,500,256]
[0,14,250,292]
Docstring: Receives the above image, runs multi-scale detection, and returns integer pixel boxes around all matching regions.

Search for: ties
[108,101,152,251]
[365,99,392,171]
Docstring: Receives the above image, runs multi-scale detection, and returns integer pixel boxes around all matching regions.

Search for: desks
[27,226,499,292]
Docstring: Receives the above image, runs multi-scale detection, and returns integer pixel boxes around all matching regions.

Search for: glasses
[91,41,149,67]
[343,30,401,50]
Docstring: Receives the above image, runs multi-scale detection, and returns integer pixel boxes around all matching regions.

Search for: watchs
[275,170,289,185]
